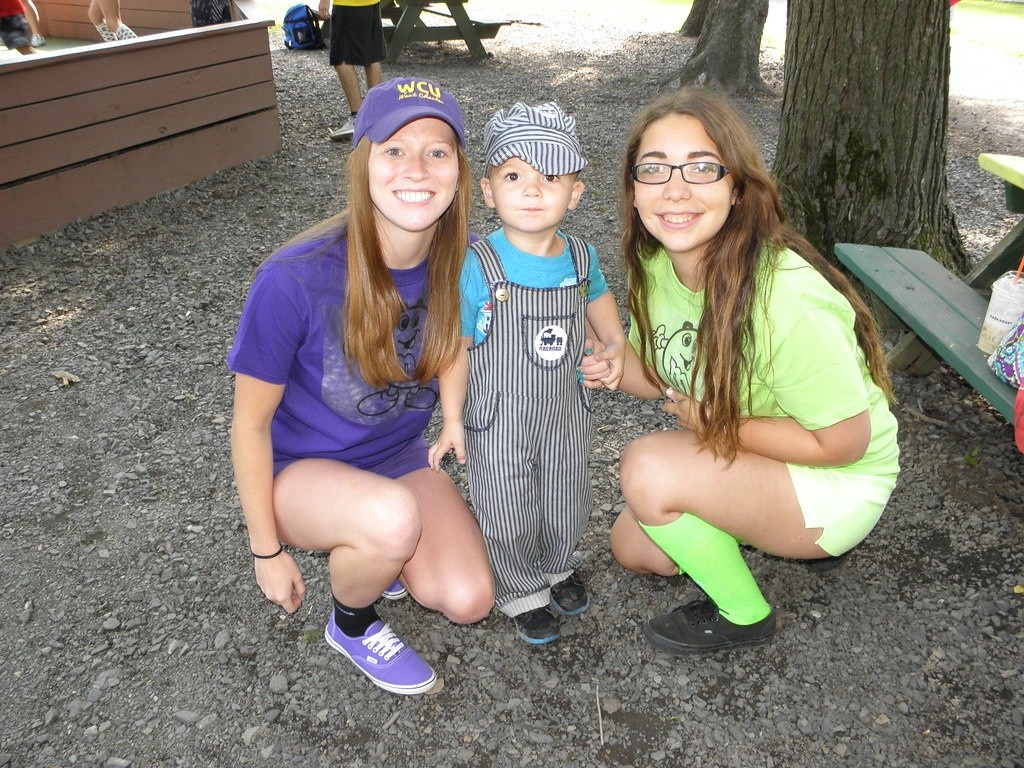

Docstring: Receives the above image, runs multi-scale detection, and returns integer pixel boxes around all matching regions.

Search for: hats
[351,76,466,152]
[484,102,586,175]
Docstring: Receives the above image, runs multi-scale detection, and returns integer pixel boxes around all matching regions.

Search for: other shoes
[30,35,45,47]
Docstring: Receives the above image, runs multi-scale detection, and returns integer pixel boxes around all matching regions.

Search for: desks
[884,152,1021,379]
[382,0,487,65]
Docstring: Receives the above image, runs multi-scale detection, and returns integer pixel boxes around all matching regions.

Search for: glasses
[629,162,731,184]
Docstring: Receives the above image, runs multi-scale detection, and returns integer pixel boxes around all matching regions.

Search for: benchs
[832,241,1018,424]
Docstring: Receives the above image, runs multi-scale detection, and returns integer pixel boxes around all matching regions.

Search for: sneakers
[515,608,558,645]
[808,552,846,573]
[643,597,778,651]
[550,573,590,614]
[329,111,362,141]
[381,577,408,600]
[324,598,437,695]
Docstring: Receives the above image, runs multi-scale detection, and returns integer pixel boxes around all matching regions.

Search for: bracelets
[252,541,282,559]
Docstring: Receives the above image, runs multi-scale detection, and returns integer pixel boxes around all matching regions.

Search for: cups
[978,270,1024,355]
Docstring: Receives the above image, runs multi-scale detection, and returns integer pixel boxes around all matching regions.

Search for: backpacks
[283,4,324,50]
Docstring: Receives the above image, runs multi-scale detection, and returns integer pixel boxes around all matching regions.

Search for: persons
[227,77,611,694]
[0,0,390,142]
[583,90,901,654]
[428,100,626,644]
